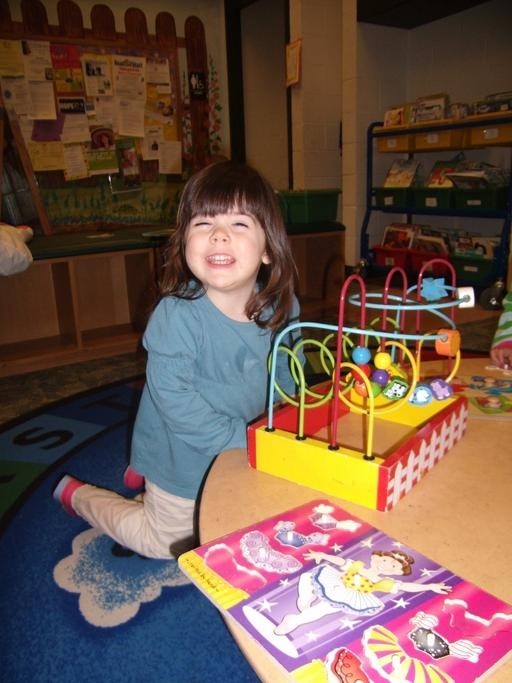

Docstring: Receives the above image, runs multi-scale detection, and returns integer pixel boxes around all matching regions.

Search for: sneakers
[123,464,145,490]
[53,475,85,517]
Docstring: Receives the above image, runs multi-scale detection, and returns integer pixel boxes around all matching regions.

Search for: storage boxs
[372,111,512,283]
[275,188,342,235]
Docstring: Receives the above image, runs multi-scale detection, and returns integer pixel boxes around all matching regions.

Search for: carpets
[1,313,502,683]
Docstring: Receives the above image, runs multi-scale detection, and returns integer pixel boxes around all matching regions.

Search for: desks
[196,358,512,683]
[1,220,346,375]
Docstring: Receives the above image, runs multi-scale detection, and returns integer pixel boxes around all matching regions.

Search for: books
[382,92,512,258]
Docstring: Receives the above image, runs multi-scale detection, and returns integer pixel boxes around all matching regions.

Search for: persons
[490,292,512,368]
[275,550,452,635]
[54,160,307,560]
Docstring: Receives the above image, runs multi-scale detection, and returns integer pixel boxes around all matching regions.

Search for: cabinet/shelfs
[361,109,512,294]
